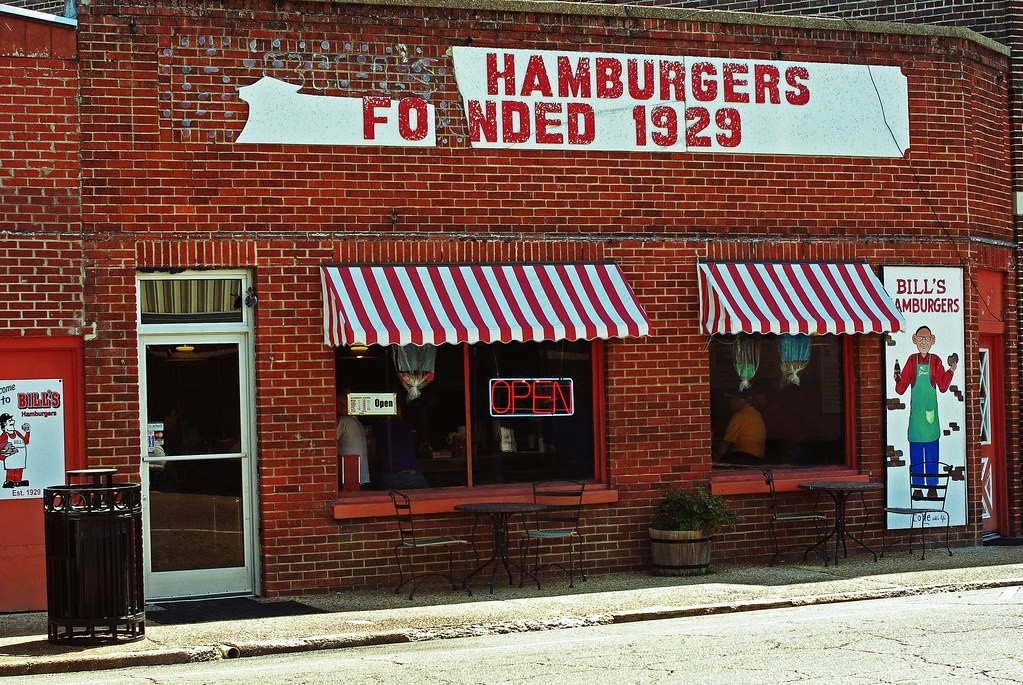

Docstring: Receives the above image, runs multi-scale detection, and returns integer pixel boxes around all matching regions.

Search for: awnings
[693,259,906,338]
[314,259,654,348]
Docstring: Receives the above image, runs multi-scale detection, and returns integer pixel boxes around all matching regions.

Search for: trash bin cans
[43,469,146,647]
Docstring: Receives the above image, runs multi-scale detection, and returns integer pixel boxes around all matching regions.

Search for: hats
[724,389,751,398]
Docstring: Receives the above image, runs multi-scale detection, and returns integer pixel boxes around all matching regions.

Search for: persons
[334,389,372,490]
[716,393,767,465]
[745,383,806,462]
[383,396,431,488]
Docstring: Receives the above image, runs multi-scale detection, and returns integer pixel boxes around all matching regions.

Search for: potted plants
[646,483,746,576]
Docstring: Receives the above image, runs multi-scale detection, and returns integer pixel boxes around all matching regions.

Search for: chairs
[878,461,954,561]
[388,488,474,602]
[518,478,589,589]
[761,467,832,569]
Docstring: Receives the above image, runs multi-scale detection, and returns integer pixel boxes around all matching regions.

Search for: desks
[452,502,550,595]
[797,481,885,568]
